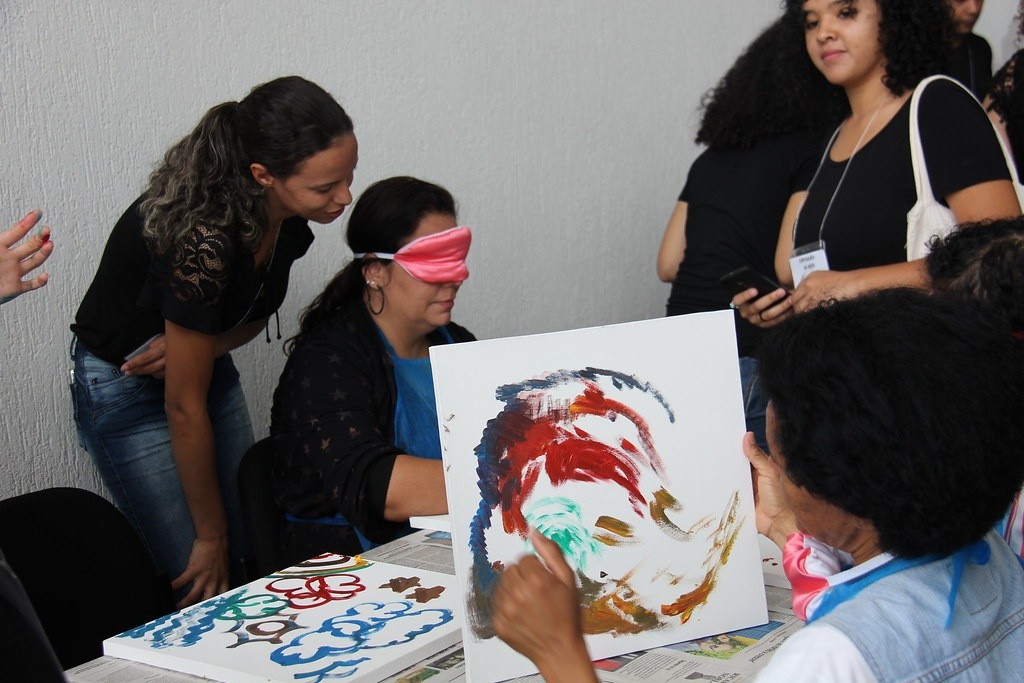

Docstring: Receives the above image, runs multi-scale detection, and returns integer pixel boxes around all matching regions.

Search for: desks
[67,528,806,683]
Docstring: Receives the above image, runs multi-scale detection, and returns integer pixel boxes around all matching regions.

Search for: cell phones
[733,267,791,310]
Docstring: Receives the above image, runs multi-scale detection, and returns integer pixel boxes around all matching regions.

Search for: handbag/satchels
[906,74,1024,262]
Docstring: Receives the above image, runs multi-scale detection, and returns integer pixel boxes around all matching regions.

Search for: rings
[759,312,766,324]
[729,300,739,310]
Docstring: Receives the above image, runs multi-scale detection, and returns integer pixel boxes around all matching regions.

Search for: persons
[730,0,1024,328]
[69,77,359,613]
[492,284,1024,683]
[0,210,54,307]
[268,176,477,588]
[655,0,1024,458]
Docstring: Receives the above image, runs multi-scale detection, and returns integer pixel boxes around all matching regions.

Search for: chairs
[0,438,274,683]
